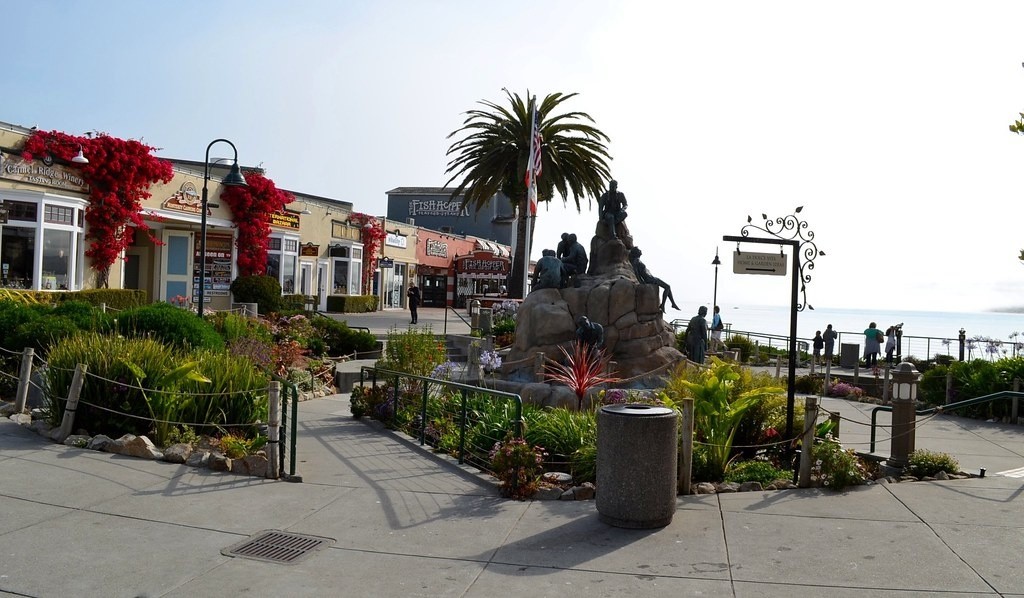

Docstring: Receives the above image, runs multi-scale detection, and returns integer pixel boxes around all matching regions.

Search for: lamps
[297,200,311,214]
[44,141,89,163]
[357,214,373,231]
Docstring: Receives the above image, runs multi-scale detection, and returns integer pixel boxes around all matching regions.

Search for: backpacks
[419,291,423,305]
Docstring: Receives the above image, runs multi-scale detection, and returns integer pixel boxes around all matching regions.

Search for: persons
[529,233,589,295]
[631,246,681,313]
[407,281,420,325]
[599,180,628,238]
[564,315,604,374]
[50,248,67,283]
[822,324,837,366]
[709,306,728,353]
[812,330,824,364]
[885,326,896,369]
[684,306,708,365]
[863,322,884,372]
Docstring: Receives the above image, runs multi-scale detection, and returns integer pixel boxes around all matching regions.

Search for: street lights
[711,245,721,319]
[198,138,250,318]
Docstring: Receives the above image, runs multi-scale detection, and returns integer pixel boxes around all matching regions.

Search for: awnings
[475,240,510,257]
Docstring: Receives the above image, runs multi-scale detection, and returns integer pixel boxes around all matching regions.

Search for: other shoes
[410,321,413,324]
[723,346,728,351]
[711,349,717,353]
[414,322,417,324]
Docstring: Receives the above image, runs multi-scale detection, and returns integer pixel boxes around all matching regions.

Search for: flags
[525,105,543,216]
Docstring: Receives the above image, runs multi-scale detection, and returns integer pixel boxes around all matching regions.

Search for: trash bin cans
[232,302,259,319]
[841,342,859,369]
[596,404,681,530]
[479,308,493,331]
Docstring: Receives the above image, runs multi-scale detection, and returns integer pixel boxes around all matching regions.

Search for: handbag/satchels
[877,333,884,343]
[717,320,723,329]
